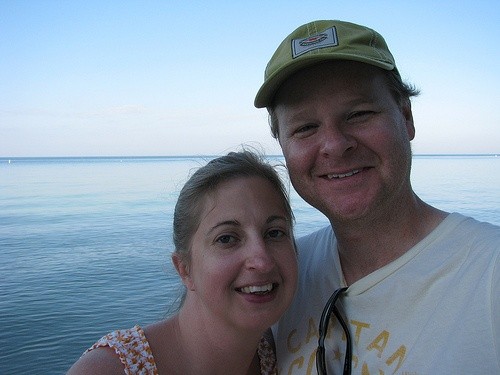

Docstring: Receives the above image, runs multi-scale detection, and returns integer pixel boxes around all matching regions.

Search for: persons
[67,152,298,374]
[253,19,500,374]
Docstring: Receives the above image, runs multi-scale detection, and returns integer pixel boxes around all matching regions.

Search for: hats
[254,20,396,108]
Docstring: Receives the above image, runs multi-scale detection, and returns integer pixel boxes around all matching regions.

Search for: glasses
[316,285,351,375]
